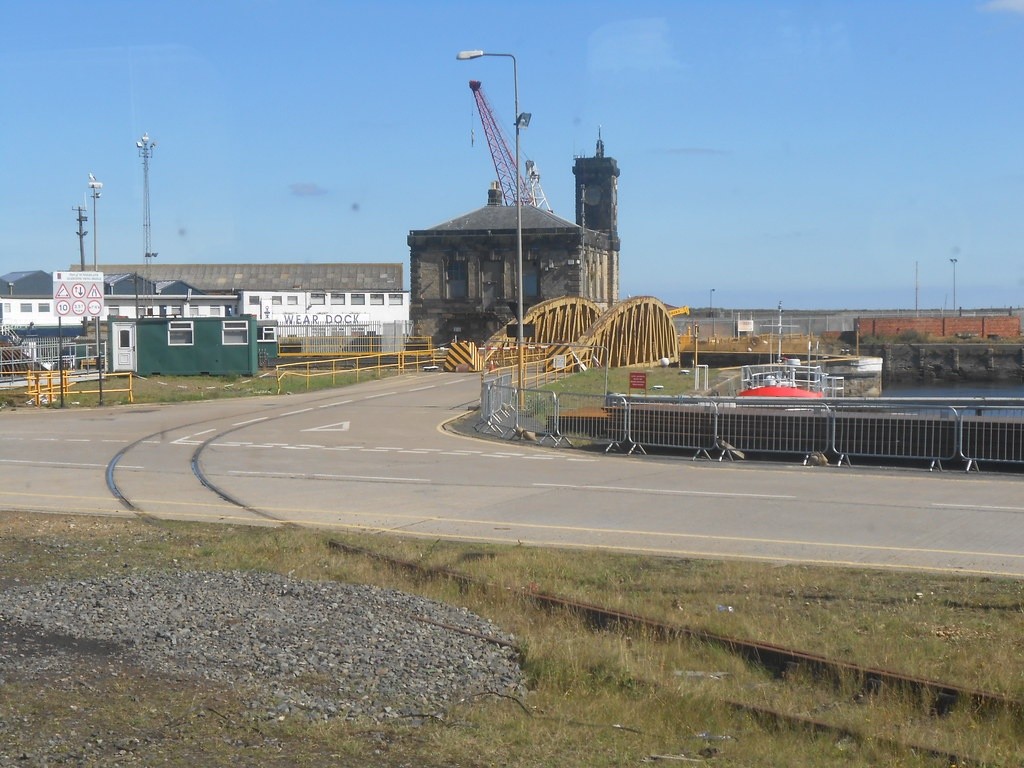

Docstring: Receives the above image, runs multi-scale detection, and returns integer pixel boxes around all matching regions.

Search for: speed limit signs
[55,300,71,315]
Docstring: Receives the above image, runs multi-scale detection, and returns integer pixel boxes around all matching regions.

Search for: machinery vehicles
[466,77,534,207]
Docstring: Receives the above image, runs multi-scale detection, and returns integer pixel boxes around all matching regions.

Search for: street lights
[455,50,527,411]
[950,257,958,312]
[135,131,157,296]
